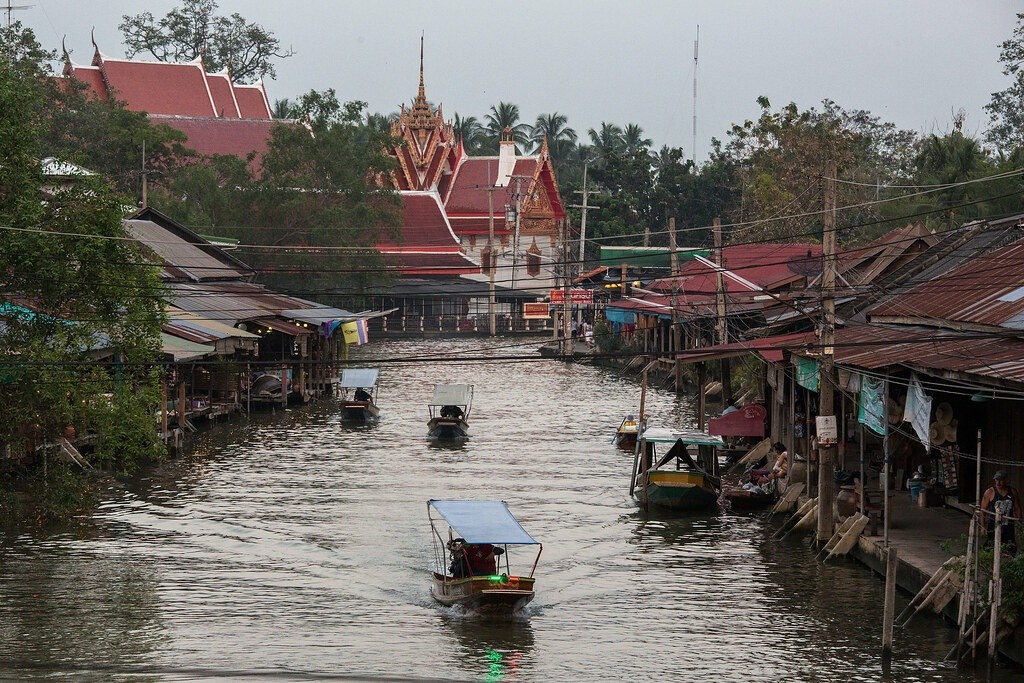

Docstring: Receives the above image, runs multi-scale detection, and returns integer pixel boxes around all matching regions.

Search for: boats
[427,498,543,613]
[615,413,651,450]
[427,382,474,448]
[339,366,381,422]
[630,369,779,517]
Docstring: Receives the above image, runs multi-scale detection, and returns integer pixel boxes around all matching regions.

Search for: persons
[794,402,818,455]
[571,317,588,338]
[354,387,373,403]
[440,405,465,419]
[979,470,1021,558]
[465,544,504,575]
[768,441,805,480]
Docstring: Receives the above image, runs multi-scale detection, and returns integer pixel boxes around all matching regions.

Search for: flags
[341,321,358,344]
[356,320,368,345]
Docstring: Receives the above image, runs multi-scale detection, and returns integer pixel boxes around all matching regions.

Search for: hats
[993,470,1008,480]
